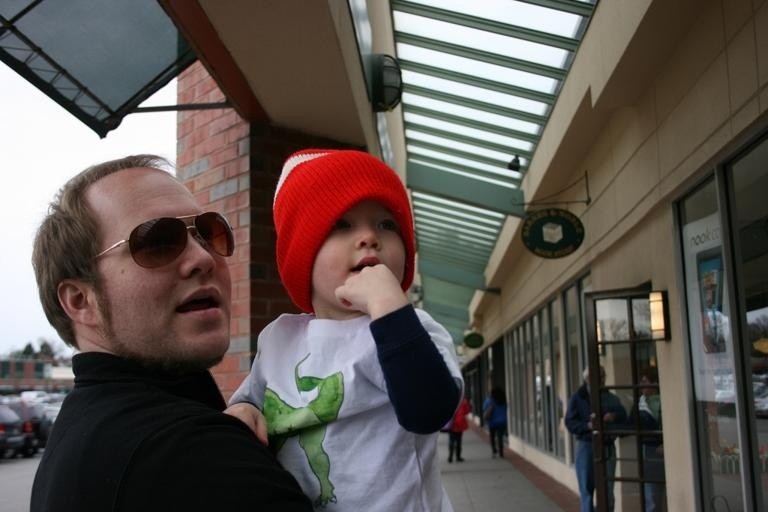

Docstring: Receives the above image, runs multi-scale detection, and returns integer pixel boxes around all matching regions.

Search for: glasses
[89,209,236,270]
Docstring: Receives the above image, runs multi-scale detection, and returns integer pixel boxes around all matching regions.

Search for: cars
[706,370,768,419]
[0,389,68,459]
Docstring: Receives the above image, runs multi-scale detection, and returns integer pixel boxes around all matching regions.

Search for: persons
[442,397,473,463]
[566,364,626,511]
[216,143,466,511]
[480,389,513,458]
[623,366,668,512]
[22,152,317,511]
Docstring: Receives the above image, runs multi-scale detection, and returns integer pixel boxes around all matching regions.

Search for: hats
[273,149,415,313]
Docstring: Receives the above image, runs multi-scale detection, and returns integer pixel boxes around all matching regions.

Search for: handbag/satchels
[483,405,494,423]
[440,415,453,433]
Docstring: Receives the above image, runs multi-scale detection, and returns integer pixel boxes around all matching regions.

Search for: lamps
[373,51,402,113]
[648,290,670,341]
